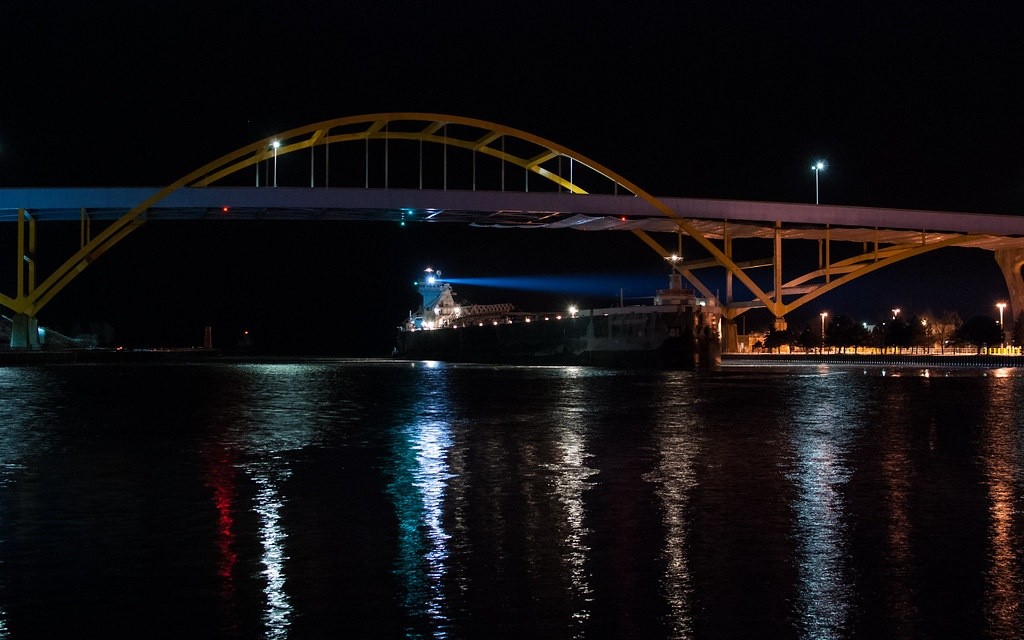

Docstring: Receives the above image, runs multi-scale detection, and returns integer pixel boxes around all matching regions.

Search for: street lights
[996,303,1006,348]
[811,160,823,205]
[273,140,280,187]
[820,312,827,348]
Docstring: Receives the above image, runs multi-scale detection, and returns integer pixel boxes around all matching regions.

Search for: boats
[393,282,725,373]
